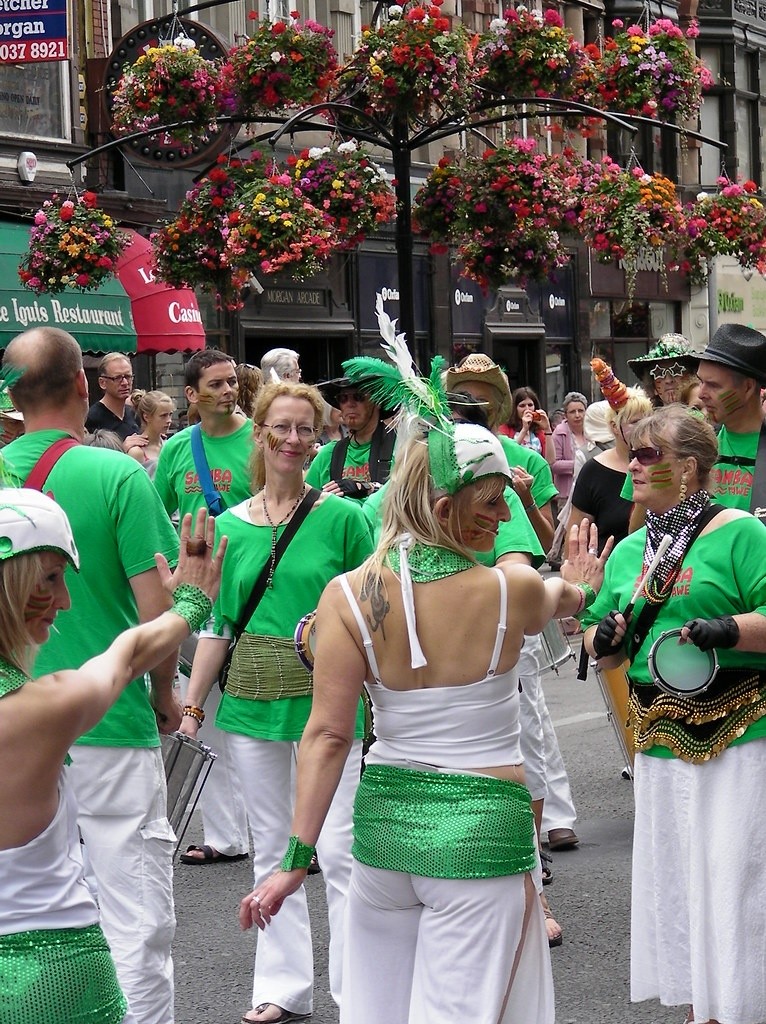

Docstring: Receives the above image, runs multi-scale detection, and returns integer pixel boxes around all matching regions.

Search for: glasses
[629,447,689,466]
[289,369,302,374]
[239,363,254,370]
[263,423,316,436]
[99,374,134,382]
[334,393,372,404]
[648,363,689,380]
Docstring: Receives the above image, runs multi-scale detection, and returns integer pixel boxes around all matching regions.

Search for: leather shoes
[548,828,579,847]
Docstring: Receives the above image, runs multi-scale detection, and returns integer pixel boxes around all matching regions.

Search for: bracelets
[525,499,536,512]
[543,432,553,435]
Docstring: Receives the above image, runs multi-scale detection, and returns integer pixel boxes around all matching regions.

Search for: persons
[0,289,766,1024]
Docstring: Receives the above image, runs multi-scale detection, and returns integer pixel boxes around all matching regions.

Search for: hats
[448,354,512,403]
[627,333,703,381]
[317,370,401,419]
[690,324,766,384]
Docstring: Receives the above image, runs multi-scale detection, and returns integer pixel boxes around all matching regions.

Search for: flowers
[112,39,234,159]
[18,189,131,295]
[148,0,765,312]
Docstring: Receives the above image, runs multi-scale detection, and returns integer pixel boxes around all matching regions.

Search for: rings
[589,549,599,555]
[252,896,262,904]
[187,538,206,556]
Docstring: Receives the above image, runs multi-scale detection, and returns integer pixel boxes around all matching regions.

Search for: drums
[293,608,318,672]
[79,725,217,848]
[647,627,721,700]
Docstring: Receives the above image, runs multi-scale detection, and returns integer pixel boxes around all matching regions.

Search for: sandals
[539,850,554,883]
[545,911,561,946]
[180,844,249,862]
[307,854,321,872]
[243,1003,313,1024]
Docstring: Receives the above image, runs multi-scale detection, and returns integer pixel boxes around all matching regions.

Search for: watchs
[371,482,381,493]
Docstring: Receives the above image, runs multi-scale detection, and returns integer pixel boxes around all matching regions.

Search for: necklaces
[262,481,305,590]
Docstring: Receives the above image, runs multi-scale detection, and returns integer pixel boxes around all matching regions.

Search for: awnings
[115,228,206,356]
[0,221,138,358]
